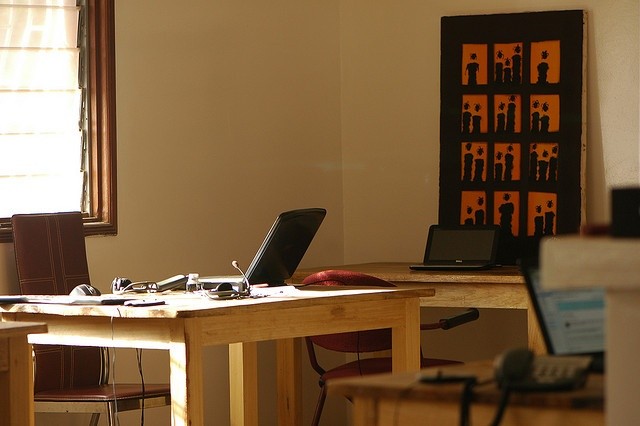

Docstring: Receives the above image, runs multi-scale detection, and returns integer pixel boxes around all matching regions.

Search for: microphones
[231,260,251,296]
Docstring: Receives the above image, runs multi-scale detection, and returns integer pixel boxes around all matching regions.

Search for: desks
[538,234,636,426]
[273,263,549,426]
[326,359,608,423]
[1,318,48,426]
[1,288,437,424]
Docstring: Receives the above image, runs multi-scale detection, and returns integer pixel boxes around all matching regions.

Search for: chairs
[301,271,481,425]
[13,208,170,426]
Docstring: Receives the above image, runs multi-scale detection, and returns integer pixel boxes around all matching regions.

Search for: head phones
[109,276,159,295]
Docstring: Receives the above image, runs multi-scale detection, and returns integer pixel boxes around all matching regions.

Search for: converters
[154,274,190,292]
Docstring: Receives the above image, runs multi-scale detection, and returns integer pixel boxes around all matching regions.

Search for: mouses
[68,282,101,296]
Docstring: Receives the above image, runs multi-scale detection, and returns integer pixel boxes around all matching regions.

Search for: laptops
[409,224,502,273]
[194,207,327,290]
